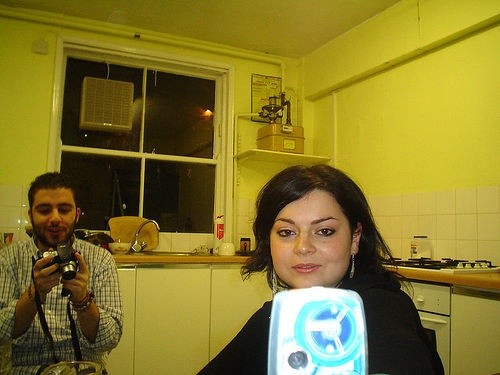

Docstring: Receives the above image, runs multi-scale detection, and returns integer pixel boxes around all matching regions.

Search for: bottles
[410,235,432,259]
[212,215,225,254]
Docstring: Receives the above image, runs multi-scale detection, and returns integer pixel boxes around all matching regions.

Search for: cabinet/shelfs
[106,264,272,375]
[450,287,500,375]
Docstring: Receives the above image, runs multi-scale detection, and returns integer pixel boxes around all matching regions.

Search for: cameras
[41,242,78,279]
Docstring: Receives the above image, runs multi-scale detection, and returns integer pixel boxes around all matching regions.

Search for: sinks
[145,252,193,256]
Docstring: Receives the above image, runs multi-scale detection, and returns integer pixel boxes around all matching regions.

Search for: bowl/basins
[217,242,235,255]
[108,242,131,254]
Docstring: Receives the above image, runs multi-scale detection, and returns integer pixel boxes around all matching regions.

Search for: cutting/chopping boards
[108,216,159,251]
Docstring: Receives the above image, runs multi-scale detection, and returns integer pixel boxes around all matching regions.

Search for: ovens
[399,278,452,375]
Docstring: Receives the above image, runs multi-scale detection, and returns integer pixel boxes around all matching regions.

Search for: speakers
[78,77,134,132]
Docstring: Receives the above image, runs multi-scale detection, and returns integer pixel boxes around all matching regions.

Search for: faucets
[135,219,160,255]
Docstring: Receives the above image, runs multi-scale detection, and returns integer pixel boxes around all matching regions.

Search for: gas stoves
[380,256,499,272]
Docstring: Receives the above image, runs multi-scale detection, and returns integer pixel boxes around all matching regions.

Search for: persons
[0,172,125,375]
[196,165,445,375]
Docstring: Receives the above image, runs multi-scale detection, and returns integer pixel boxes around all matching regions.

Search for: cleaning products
[214,214,225,255]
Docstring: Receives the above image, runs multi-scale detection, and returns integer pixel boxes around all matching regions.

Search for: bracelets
[70,291,95,311]
